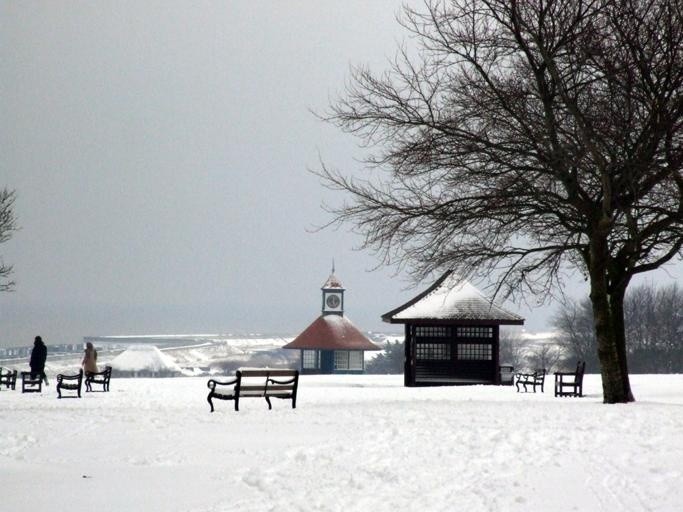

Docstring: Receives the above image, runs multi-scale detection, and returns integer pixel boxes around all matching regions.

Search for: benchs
[207,370,299,413]
[57,364,112,400]
[516,368,546,393]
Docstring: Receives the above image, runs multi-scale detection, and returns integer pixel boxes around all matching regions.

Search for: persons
[29,336,49,387]
[82,342,98,372]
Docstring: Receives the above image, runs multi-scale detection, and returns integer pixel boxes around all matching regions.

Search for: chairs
[553,361,586,398]
[20,371,43,393]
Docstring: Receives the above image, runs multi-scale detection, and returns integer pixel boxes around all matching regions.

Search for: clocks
[325,293,342,311]
